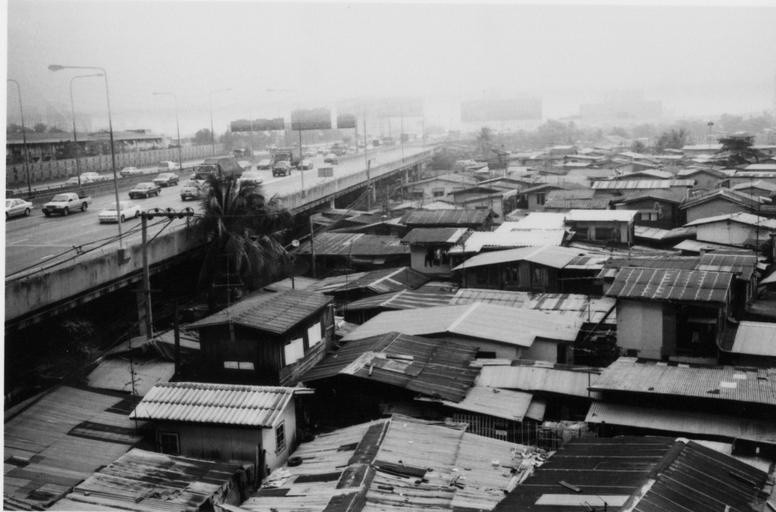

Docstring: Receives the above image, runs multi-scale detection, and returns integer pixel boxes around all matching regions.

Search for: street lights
[47,62,123,250]
[152,83,302,172]
[66,71,105,189]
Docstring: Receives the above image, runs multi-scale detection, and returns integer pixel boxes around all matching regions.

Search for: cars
[127,181,162,200]
[119,166,144,177]
[373,131,433,148]
[156,161,177,170]
[297,159,314,171]
[303,139,369,163]
[98,201,142,224]
[179,155,253,201]
[65,171,106,187]
[5,197,34,219]
[152,172,180,187]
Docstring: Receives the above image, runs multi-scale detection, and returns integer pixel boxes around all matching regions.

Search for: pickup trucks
[42,192,92,216]
